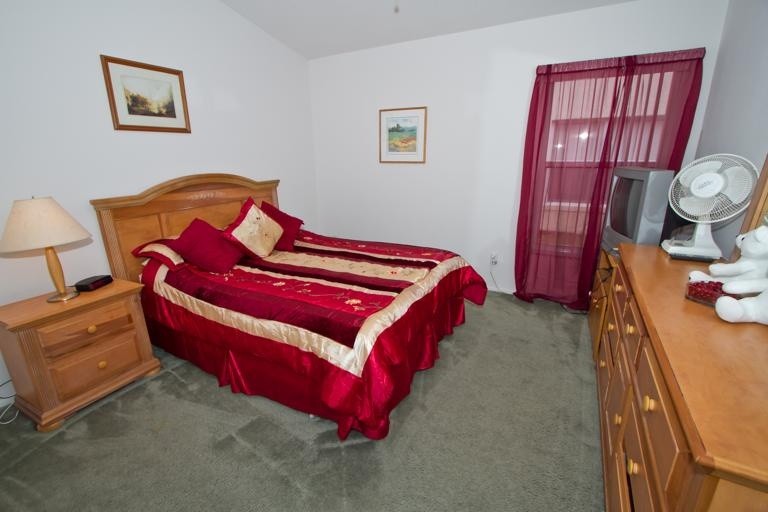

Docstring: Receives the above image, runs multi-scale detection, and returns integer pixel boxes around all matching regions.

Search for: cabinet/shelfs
[588,245,768,511]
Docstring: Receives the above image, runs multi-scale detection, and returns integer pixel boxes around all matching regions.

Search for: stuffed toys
[687,224,768,284]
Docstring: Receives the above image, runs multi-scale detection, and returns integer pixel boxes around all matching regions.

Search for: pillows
[129,197,303,274]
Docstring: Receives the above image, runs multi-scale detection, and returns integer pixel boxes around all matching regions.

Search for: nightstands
[2,279,160,433]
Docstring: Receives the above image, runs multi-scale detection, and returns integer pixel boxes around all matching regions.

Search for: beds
[88,171,469,441]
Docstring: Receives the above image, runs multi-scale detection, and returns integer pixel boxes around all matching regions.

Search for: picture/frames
[379,107,427,164]
[100,54,191,133]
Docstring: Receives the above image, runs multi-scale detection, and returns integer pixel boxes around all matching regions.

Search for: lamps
[2,196,93,305]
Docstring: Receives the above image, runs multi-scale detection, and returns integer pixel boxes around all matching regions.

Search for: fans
[660,152,757,264]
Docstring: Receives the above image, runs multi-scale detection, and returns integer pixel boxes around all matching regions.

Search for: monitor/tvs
[600,166,698,257]
